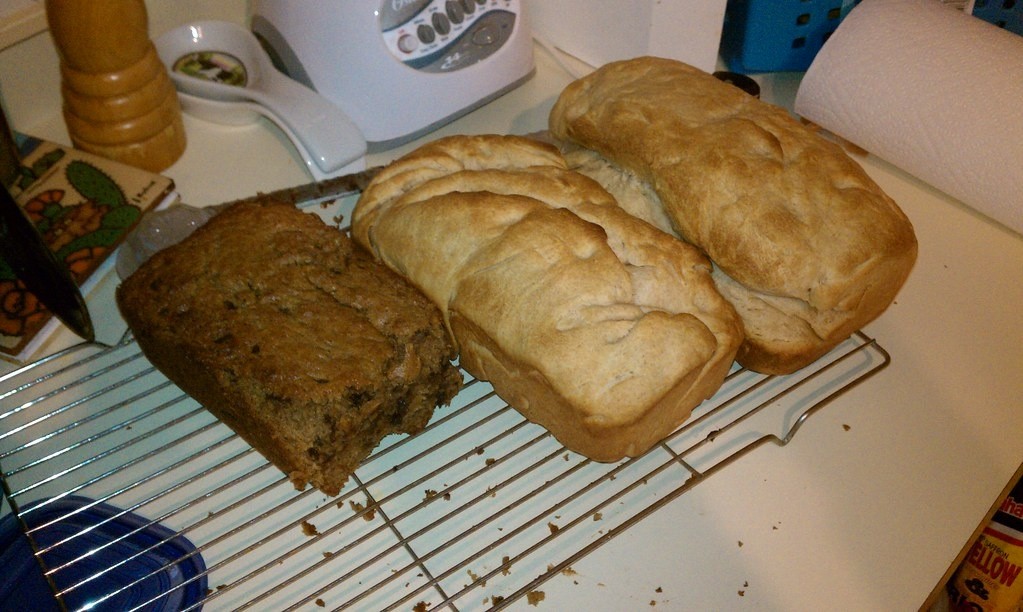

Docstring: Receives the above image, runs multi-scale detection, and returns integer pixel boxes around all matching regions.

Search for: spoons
[155,18,368,184]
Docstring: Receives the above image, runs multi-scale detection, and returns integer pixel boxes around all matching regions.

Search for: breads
[113,197,465,495]
[549,57,919,376]
[350,134,745,462]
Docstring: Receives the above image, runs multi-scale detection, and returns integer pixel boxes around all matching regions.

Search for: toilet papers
[791,0,1023,241]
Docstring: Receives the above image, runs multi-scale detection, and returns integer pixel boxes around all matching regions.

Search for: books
[0,130,180,365]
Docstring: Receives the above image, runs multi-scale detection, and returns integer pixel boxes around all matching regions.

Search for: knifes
[0,180,95,343]
